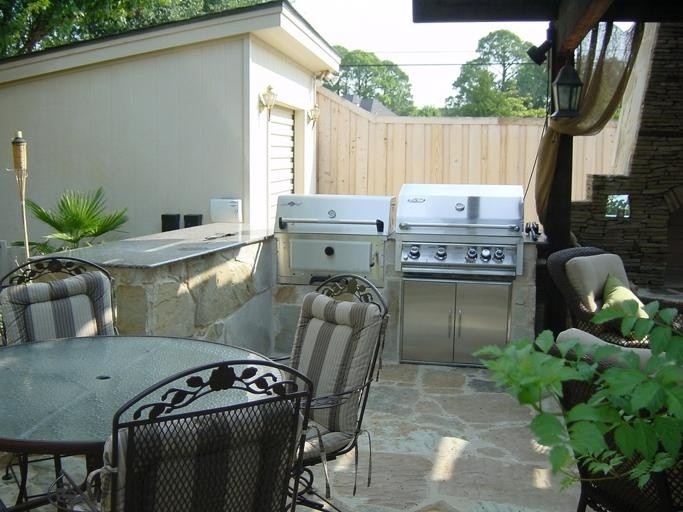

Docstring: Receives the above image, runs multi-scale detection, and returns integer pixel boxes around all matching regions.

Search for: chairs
[545,246,681,350]
[533,327,682,510]
[45,359,314,512]
[0,255,119,512]
[271,273,390,512]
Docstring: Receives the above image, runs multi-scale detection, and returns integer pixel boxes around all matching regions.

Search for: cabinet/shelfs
[399,277,511,368]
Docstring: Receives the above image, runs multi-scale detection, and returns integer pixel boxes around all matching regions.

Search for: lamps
[306,103,320,129]
[257,85,278,121]
[549,48,584,119]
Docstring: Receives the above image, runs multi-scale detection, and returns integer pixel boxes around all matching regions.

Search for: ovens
[274,233,383,288]
